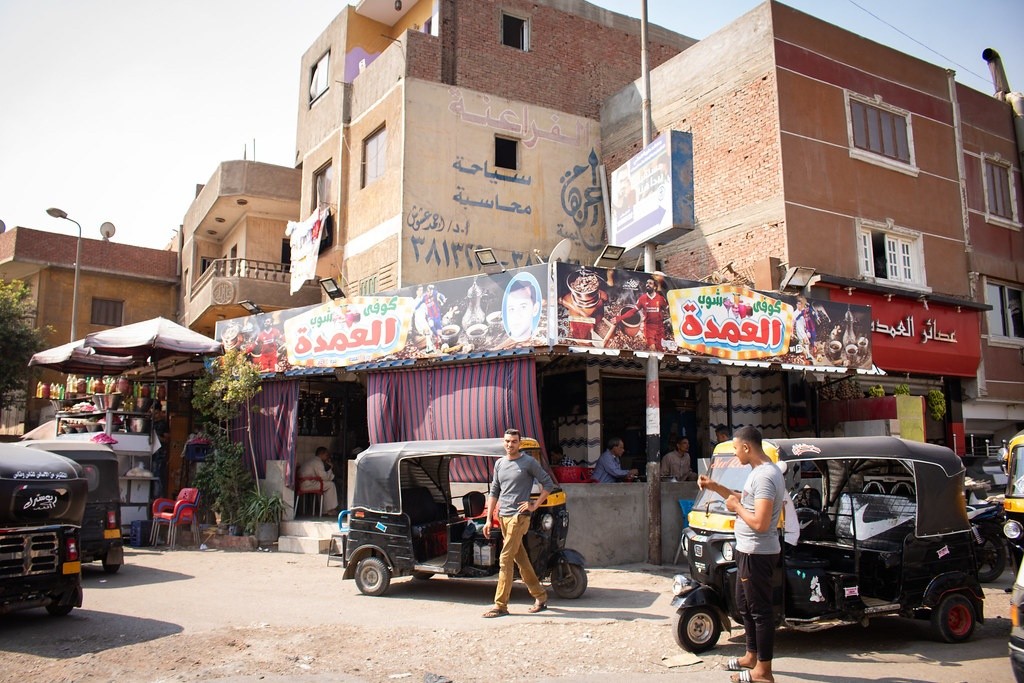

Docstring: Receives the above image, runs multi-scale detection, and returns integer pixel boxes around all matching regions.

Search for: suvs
[1,441,90,618]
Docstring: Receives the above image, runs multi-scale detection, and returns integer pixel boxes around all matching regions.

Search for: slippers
[722,658,753,671]
[482,608,509,618]
[729,670,774,683]
[528,603,548,613]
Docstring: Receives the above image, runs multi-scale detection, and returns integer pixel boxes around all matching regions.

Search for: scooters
[965,503,1007,584]
[14,438,125,575]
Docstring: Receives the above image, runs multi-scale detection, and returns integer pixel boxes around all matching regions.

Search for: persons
[144,399,170,497]
[412,286,435,353]
[590,438,638,483]
[714,426,732,443]
[795,303,821,358]
[482,429,554,618]
[297,447,341,517]
[697,426,784,683]
[507,280,540,341]
[415,285,447,344]
[792,300,812,360]
[610,278,669,352]
[660,437,697,482]
[245,317,286,372]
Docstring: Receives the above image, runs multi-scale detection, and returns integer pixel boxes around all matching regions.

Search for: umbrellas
[28,338,147,378]
[85,316,226,474]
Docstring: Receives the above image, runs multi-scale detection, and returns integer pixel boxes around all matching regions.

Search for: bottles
[843,304,856,345]
[35,372,166,401]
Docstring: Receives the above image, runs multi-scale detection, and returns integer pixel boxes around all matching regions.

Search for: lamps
[238,299,266,316]
[394,0,402,11]
[320,277,346,300]
[594,244,626,270]
[474,248,506,274]
[780,266,817,295]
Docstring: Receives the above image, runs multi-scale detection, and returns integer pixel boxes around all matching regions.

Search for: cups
[618,304,642,337]
[250,351,263,365]
[566,269,601,308]
[568,315,596,341]
[437,324,460,346]
[466,311,507,345]
[221,326,241,351]
[828,339,842,360]
[858,335,868,358]
[845,342,858,361]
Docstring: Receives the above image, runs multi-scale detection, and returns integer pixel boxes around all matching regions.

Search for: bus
[1001,429,1024,578]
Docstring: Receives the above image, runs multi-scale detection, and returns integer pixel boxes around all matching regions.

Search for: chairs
[463,491,502,540]
[673,500,695,565]
[795,486,833,539]
[148,488,201,550]
[295,477,324,519]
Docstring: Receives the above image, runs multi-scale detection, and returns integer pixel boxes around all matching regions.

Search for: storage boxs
[186,443,214,461]
[131,520,152,547]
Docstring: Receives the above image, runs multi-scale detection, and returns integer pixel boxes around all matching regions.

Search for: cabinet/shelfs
[153,415,189,499]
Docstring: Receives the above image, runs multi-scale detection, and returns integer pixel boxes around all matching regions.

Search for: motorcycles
[337,438,588,600]
[667,435,987,657]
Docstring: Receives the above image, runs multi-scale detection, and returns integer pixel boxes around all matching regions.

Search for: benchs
[401,486,458,538]
[802,492,917,567]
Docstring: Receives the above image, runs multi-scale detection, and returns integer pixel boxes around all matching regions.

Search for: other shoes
[321,508,338,517]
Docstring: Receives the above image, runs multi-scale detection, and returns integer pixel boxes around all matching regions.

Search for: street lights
[46,208,82,349]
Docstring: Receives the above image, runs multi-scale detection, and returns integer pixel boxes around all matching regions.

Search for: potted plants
[210,496,223,525]
[238,486,288,541]
